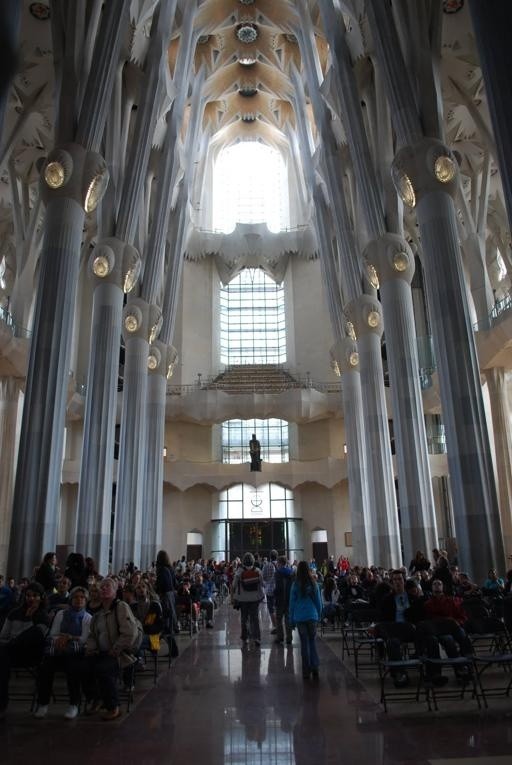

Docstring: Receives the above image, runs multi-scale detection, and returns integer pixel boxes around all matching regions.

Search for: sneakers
[64,705,78,718]
[103,707,120,720]
[35,706,47,718]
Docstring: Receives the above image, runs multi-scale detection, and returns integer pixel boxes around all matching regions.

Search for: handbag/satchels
[129,618,143,652]
[42,639,78,660]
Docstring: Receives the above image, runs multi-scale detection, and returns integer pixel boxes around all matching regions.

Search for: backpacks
[241,566,260,592]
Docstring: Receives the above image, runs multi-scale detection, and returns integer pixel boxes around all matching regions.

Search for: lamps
[249,432,265,473]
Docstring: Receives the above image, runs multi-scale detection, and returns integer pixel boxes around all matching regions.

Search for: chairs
[1,572,230,714]
[318,602,512,715]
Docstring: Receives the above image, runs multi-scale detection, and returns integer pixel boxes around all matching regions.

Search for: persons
[0,548,512,720]
[250,433,262,471]
